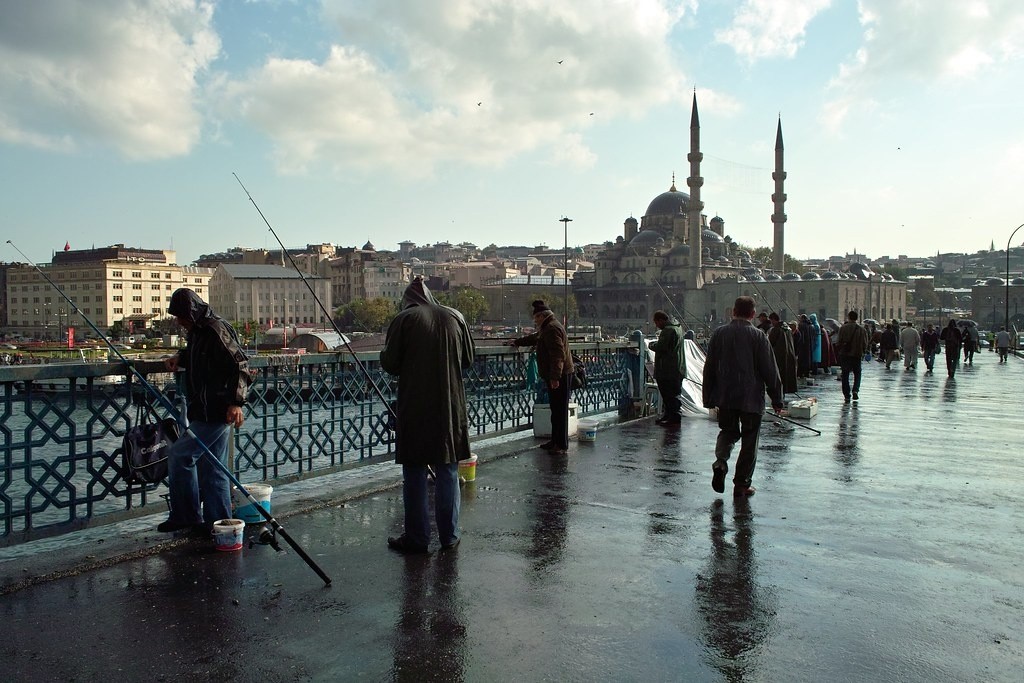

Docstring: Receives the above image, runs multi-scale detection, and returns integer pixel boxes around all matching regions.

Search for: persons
[961,322,978,363]
[881,324,897,368]
[864,324,885,352]
[900,322,920,370]
[940,319,963,379]
[158,288,251,533]
[988,331,995,351]
[921,324,938,371]
[648,310,687,427]
[702,296,783,497]
[892,319,899,341]
[755,312,839,380]
[507,300,575,452]
[379,277,476,555]
[996,326,1011,362]
[836,311,867,400]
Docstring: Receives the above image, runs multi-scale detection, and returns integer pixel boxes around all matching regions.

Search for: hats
[756,312,768,318]
[532,300,551,315]
[788,320,797,326]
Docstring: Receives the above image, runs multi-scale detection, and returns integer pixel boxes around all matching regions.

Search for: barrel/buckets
[831,366,841,375]
[577,419,599,441]
[837,370,842,381]
[233,484,273,523]
[817,368,824,376]
[849,374,853,380]
[211,519,245,551]
[866,355,871,361]
[458,453,478,482]
[806,378,814,386]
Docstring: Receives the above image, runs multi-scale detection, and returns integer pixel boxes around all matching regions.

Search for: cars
[29,337,86,343]
[470,325,535,335]
[979,340,990,345]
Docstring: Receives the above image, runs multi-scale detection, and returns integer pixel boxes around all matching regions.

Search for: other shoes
[964,356,968,362]
[911,365,915,369]
[845,396,850,400]
[388,533,427,551]
[192,522,212,534]
[886,366,890,369]
[540,441,567,450]
[445,536,460,547]
[158,509,202,532]
[655,417,667,423]
[660,420,681,426]
[853,392,858,399]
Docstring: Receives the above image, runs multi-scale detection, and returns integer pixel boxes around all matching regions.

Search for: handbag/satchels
[935,345,941,354]
[122,395,181,485]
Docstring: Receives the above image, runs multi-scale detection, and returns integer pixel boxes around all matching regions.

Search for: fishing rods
[864,347,886,364]
[751,265,801,322]
[729,251,775,313]
[6,238,333,586]
[630,246,708,356]
[231,170,438,481]
[620,339,822,436]
[669,296,803,400]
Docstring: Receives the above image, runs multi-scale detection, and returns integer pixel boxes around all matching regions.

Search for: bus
[118,335,146,344]
[567,326,602,339]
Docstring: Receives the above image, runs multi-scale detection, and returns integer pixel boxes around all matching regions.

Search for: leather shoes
[734,486,755,495]
[712,463,726,493]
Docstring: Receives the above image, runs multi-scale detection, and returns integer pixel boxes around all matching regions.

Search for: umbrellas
[958,320,978,328]
[862,319,880,326]
[825,318,842,331]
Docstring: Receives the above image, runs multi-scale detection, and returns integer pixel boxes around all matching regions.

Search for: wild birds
[477,102,481,107]
[590,113,593,116]
[558,61,563,64]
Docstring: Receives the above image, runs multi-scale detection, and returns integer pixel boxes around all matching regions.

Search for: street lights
[283,298,287,348]
[233,300,238,322]
[559,215,573,334]
[989,295,995,323]
